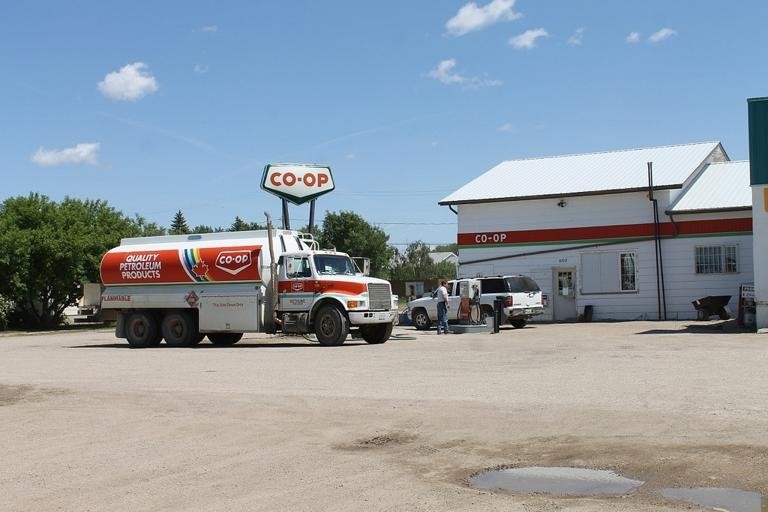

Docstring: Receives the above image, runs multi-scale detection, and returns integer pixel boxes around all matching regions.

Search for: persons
[437,280,449,334]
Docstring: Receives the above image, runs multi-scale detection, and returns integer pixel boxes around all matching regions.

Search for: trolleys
[692,294,732,320]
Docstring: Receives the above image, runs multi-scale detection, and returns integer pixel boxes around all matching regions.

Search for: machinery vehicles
[96,211,400,347]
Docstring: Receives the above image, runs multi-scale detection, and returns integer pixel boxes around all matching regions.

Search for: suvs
[405,273,544,331]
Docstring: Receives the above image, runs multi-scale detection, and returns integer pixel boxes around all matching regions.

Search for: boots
[437,329,442,335]
[444,329,454,334]
